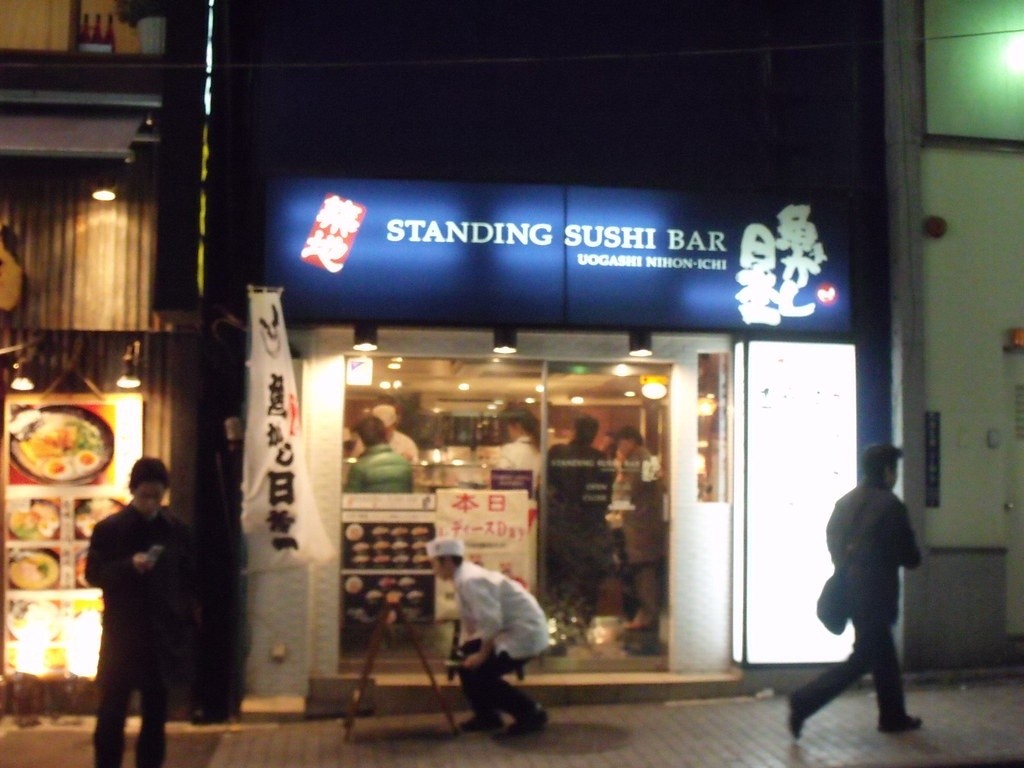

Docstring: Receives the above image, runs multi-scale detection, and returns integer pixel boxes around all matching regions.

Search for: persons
[345,402,666,630]
[424,536,553,739]
[787,441,927,737]
[84,455,198,767]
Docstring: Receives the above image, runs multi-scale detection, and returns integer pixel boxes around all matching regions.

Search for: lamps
[10,352,35,394]
[627,333,654,357]
[117,345,140,389]
[352,323,381,355]
[490,325,520,357]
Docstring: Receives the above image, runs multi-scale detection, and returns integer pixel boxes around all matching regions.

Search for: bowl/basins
[10,403,116,486]
[73,545,94,589]
[9,500,61,540]
[9,547,61,590]
[76,497,126,540]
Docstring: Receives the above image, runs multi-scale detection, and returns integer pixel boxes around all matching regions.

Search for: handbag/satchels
[817,569,849,635]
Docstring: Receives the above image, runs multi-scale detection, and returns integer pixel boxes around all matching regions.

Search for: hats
[609,425,642,445]
[865,444,904,461]
[426,538,464,558]
[372,404,397,428]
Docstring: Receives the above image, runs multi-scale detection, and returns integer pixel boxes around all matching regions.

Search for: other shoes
[789,694,803,740]
[879,717,922,731]
[508,711,548,734]
[621,618,654,628]
[461,715,504,731]
